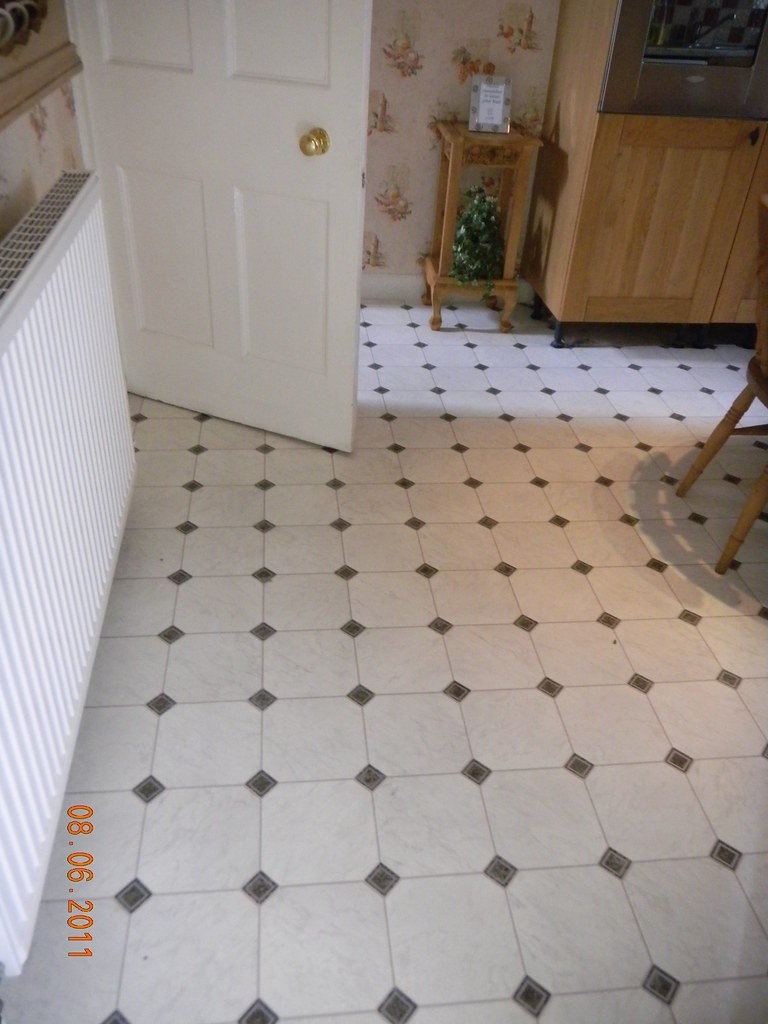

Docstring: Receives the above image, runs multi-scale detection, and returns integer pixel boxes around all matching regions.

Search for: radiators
[0,167,135,979]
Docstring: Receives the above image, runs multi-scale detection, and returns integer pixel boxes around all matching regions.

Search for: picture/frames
[469,75,513,134]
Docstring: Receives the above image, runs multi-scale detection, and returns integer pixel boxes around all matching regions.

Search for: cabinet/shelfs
[527,0,768,348]
[422,121,544,332]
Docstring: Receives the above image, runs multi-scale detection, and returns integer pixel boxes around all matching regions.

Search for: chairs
[676,191,768,574]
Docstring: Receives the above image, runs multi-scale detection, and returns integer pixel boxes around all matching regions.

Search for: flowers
[452,183,504,302]
